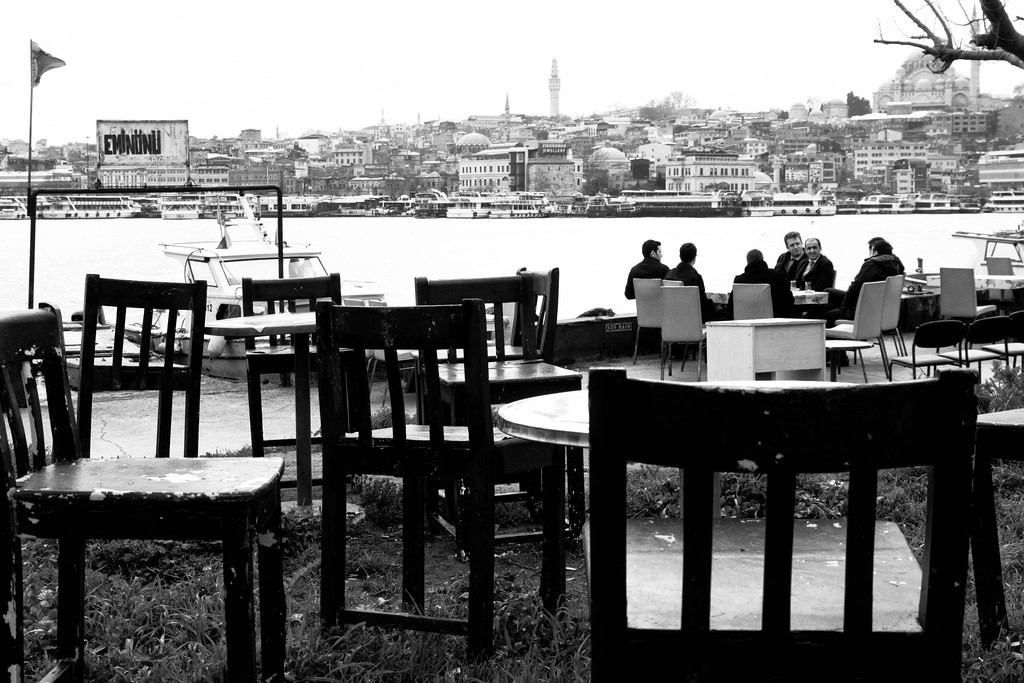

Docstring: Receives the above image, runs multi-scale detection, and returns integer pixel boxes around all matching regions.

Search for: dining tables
[701,286,830,306]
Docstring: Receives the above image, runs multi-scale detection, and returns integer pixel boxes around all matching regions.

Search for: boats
[904,226,1024,314]
[126,194,386,386]
[1,175,1023,222]
[53,309,189,396]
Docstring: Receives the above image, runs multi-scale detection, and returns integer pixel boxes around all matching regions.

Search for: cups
[790,280,796,293]
[805,282,811,292]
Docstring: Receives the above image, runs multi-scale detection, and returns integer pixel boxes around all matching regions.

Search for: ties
[802,262,814,279]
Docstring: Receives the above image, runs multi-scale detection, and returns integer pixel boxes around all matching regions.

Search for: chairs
[0,307,289,683]
[825,280,890,383]
[65,273,208,457]
[584,365,984,682]
[864,271,908,358]
[660,280,707,372]
[342,299,420,410]
[413,272,588,564]
[310,298,572,667]
[659,285,707,383]
[888,320,965,382]
[631,278,673,365]
[833,274,904,373]
[732,283,774,320]
[974,407,1024,656]
[935,267,998,354]
[241,272,365,496]
[409,266,562,426]
[978,309,1024,386]
[932,314,1012,389]
[984,256,1021,316]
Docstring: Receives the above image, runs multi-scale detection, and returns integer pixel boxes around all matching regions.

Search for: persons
[774,231,809,289]
[871,240,906,282]
[725,248,796,322]
[624,238,670,299]
[811,236,898,368]
[664,241,726,327]
[780,235,834,330]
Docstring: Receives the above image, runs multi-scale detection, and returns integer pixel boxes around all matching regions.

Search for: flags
[30,38,67,85]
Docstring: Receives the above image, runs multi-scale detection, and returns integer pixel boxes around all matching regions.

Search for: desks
[925,274,1024,307]
[204,311,369,536]
[496,378,869,454]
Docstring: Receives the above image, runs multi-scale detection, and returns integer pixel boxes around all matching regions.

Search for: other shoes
[840,356,849,366]
